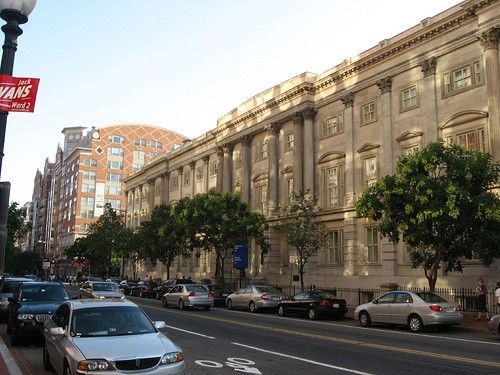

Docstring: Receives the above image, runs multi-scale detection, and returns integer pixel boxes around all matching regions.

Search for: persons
[49,273,55,281]
[181,276,192,283]
[474,278,490,320]
[82,274,85,279]
[69,274,73,285]
[145,275,152,282]
[494,282,500,307]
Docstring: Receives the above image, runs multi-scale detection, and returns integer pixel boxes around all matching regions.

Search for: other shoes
[473,317,480,321]
[483,317,490,320]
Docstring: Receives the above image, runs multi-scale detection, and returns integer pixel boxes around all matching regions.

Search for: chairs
[107,312,128,331]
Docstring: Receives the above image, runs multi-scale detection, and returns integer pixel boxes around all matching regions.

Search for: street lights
[0,1,37,174]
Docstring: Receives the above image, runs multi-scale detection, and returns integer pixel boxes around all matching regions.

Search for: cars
[78,281,125,300]
[43,299,185,375]
[225,284,288,313]
[0,275,71,346]
[163,283,231,311]
[275,291,348,321]
[488,314,500,342]
[84,277,159,298]
[354,291,464,333]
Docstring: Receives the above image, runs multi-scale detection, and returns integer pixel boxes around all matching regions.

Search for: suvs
[156,278,192,300]
